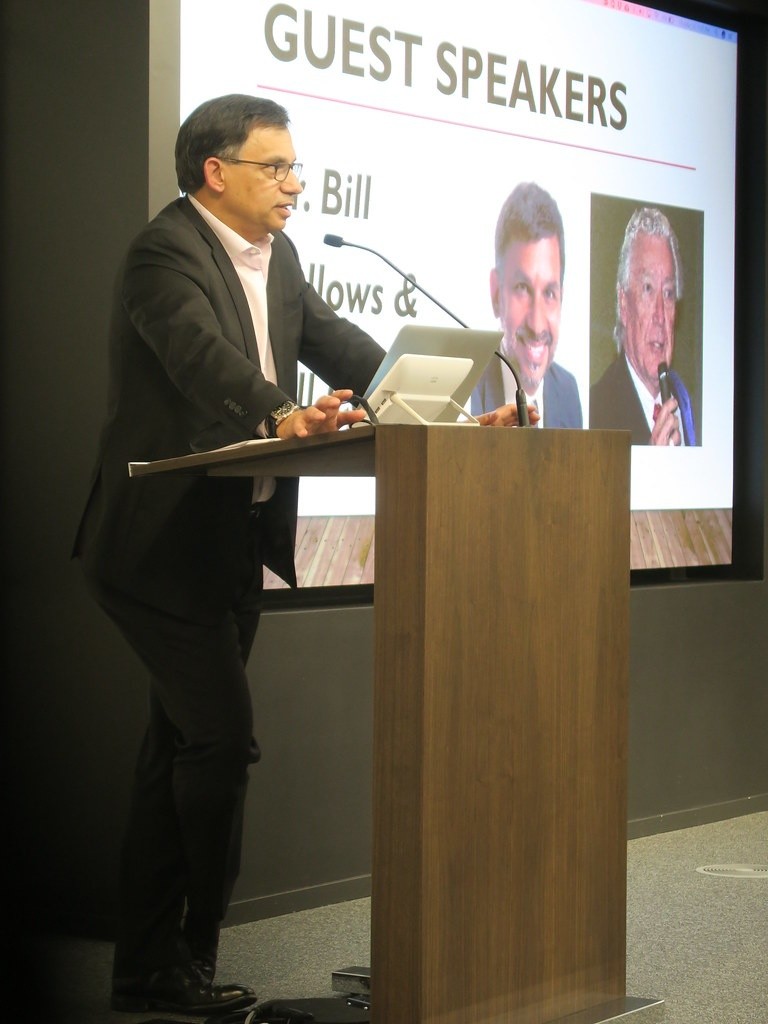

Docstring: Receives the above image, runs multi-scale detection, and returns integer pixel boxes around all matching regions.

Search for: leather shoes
[175,933,219,988]
[110,950,258,1013]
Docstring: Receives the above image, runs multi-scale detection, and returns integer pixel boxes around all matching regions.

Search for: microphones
[657,362,671,404]
[323,234,534,430]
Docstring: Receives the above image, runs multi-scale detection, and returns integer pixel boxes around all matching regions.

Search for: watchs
[263,398,302,439]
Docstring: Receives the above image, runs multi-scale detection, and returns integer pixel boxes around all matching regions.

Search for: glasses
[214,155,304,181]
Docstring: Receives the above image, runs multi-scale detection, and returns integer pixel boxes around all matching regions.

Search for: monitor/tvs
[348,325,504,424]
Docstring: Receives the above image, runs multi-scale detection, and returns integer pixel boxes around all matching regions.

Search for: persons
[467,182,584,433]
[67,95,538,1015]
[590,201,702,447]
